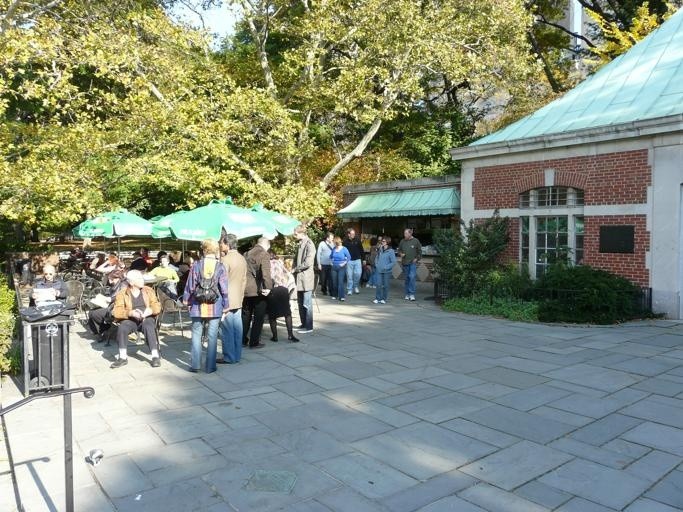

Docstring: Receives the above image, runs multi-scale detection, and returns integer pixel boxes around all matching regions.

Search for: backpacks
[191,261,223,304]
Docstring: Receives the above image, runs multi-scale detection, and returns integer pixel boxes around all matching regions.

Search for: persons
[15,226,423,372]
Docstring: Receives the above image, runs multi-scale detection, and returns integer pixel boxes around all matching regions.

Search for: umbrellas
[72,199,303,262]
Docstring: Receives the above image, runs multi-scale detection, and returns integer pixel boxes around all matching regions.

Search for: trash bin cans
[19,304,77,399]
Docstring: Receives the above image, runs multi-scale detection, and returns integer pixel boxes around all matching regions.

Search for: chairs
[31,258,188,360]
[312,274,320,313]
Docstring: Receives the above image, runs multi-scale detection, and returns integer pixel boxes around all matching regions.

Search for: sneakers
[330,287,415,304]
[215,358,229,364]
[188,368,199,373]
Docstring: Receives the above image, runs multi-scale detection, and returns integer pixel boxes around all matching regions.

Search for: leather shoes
[108,356,129,369]
[150,356,160,367]
[241,325,315,349]
